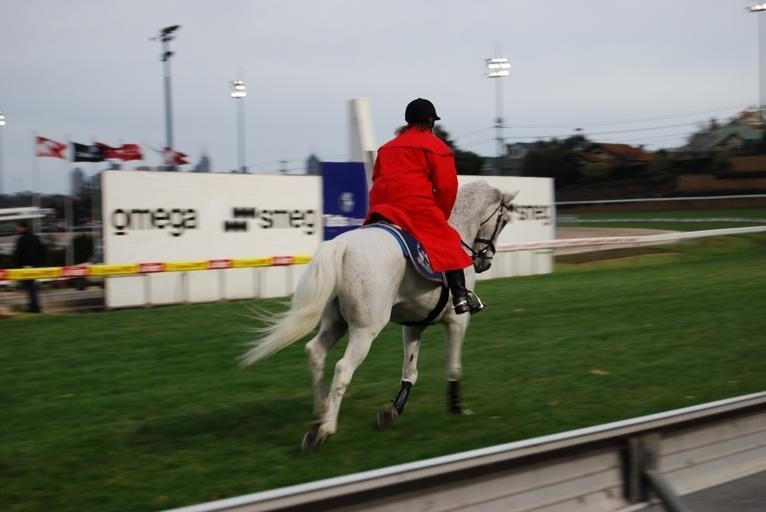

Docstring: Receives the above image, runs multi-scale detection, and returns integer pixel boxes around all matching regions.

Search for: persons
[363,97,486,313]
[12,223,47,315]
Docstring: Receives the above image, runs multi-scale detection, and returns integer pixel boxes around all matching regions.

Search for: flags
[34,136,190,167]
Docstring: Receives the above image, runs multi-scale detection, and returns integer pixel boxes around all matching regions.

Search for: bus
[0,207,59,254]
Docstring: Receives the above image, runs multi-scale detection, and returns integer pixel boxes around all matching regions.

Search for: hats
[405,97,441,122]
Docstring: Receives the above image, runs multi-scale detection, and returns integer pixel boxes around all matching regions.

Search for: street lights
[231,77,247,172]
[486,58,511,157]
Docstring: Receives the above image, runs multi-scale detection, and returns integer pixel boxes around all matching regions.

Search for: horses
[228,180,518,453]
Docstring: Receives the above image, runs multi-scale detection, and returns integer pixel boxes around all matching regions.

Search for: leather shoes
[453,294,487,314]
